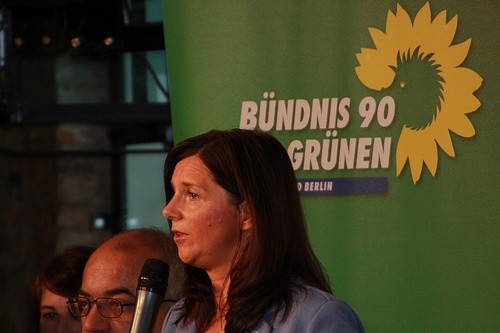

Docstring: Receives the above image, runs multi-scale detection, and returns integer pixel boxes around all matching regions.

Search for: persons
[160,127,364,333]
[27,243,95,333]
[65,226,186,333]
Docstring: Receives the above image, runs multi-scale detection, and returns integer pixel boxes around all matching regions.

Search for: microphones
[128,258,170,333]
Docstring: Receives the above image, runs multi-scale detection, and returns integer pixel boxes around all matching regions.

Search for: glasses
[66,296,179,319]
